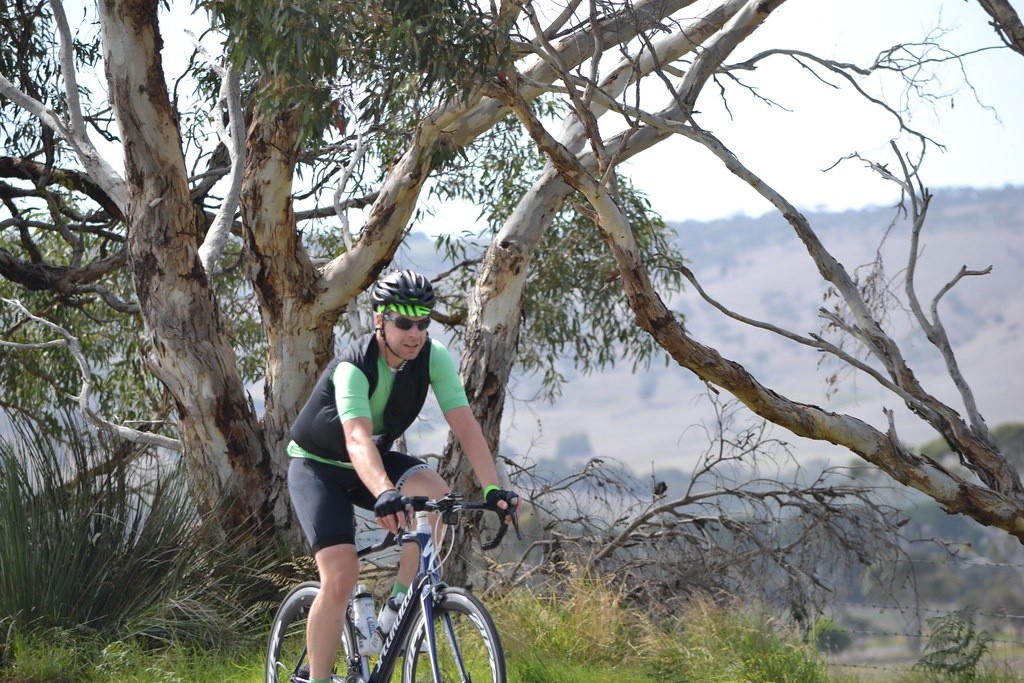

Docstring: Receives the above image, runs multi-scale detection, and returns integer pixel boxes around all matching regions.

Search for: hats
[376,286,432,317]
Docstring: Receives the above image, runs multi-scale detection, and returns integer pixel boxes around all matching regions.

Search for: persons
[285,268,524,682]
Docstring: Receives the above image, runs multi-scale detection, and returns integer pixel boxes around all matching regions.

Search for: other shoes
[402,632,429,652]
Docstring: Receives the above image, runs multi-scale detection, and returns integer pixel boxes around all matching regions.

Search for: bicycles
[265,489,523,683]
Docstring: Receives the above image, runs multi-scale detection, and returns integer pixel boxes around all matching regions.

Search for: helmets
[370,270,434,312]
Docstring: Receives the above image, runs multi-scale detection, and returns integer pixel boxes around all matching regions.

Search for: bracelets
[483,485,499,498]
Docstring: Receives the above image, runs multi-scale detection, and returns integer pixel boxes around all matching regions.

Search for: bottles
[369,592,404,653]
[351,584,378,655]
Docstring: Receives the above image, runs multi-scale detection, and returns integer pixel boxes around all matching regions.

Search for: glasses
[385,314,430,330]
